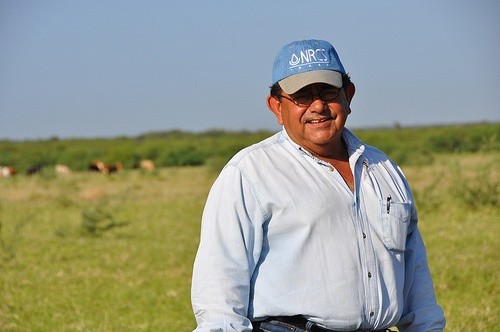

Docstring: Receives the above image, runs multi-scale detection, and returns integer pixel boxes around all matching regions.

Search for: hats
[272,39,345,95]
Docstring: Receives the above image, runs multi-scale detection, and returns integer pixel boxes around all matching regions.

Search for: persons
[190,37,449,331]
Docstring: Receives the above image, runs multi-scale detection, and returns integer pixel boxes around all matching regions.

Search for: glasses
[279,85,344,104]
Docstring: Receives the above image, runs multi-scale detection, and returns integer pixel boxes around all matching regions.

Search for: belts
[270,315,336,332]
